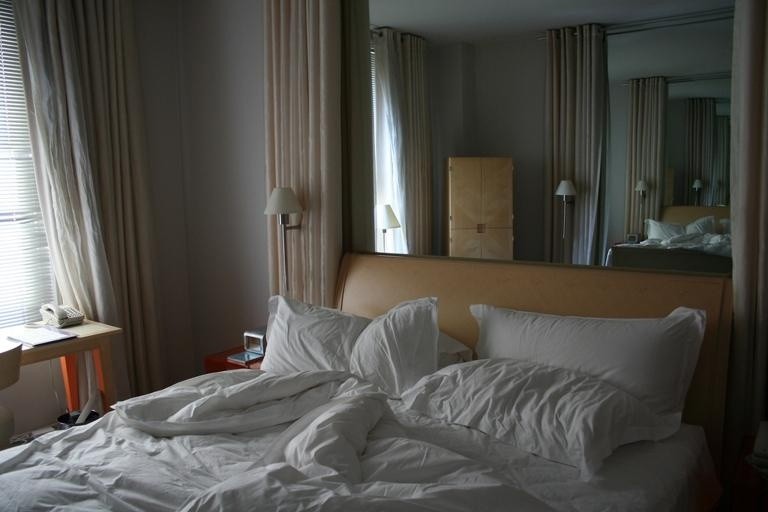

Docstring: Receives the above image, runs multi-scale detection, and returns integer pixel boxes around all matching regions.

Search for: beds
[0,251,732,511]
[605,205,732,273]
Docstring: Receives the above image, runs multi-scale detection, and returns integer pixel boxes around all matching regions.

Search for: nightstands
[202,346,261,374]
[732,435,768,511]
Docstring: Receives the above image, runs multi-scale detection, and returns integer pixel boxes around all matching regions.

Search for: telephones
[38,301,85,330]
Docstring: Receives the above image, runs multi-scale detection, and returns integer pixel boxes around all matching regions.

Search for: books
[7,328,79,346]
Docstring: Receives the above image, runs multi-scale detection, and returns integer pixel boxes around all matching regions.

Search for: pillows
[643,215,716,241]
[405,355,678,474]
[261,295,439,398]
[468,304,706,429]
[718,218,730,233]
[440,328,474,365]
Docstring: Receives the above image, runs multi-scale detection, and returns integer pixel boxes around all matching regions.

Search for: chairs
[0,345,24,444]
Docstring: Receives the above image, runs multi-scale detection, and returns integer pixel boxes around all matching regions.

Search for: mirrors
[341,0,736,278]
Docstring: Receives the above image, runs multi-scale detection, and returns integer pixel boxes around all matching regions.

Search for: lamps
[375,205,401,253]
[265,184,305,294]
[690,179,705,206]
[552,179,577,239]
[633,179,652,207]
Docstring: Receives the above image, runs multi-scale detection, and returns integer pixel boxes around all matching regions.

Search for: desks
[0,314,125,414]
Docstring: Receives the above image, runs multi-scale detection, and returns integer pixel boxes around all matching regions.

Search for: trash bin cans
[56,410,99,430]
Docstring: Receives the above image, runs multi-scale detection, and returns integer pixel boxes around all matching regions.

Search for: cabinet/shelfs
[445,156,516,262]
